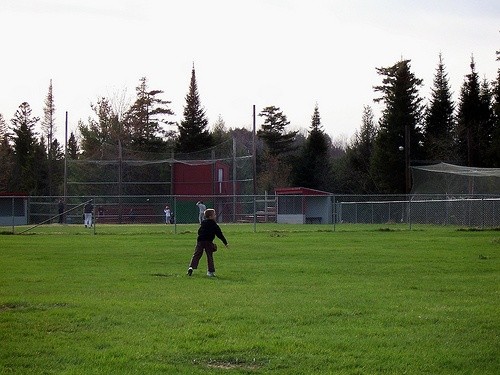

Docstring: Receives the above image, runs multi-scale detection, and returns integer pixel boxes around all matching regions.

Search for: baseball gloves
[212,243,217,253]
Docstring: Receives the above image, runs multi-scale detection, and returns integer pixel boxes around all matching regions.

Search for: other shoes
[85,225,87,228]
[89,227,91,228]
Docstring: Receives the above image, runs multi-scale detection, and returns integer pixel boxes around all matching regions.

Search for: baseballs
[399,146,403,151]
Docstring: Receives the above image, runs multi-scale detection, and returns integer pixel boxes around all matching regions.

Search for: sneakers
[188,267,193,276]
[207,271,216,276]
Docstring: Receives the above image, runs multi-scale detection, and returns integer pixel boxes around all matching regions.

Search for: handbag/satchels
[208,241,217,251]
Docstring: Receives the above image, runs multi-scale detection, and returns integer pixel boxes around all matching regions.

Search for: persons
[187,209,229,277]
[84,201,93,229]
[164,206,172,224]
[196,200,206,225]
[97,204,108,221]
[58,200,64,224]
[129,208,135,224]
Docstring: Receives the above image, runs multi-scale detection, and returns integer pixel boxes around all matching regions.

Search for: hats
[201,200,203,202]
[166,205,170,208]
